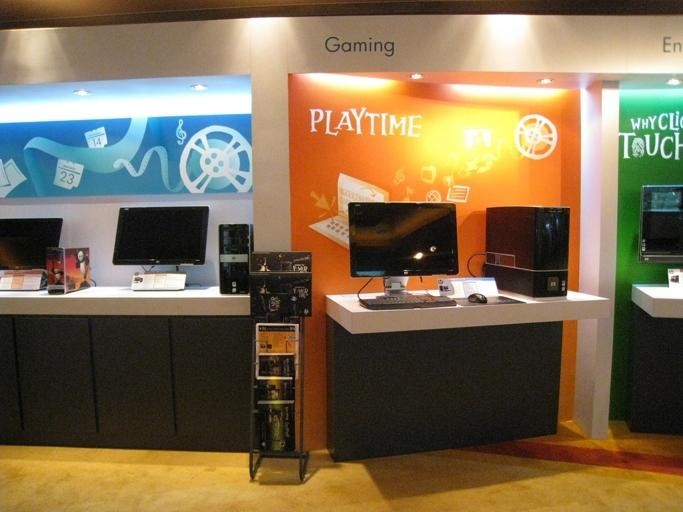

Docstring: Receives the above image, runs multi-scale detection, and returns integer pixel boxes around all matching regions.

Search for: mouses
[468,293,487,303]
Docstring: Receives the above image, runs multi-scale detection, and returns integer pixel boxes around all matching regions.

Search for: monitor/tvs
[112,206,209,272]
[0,218,63,269]
[639,185,683,265]
[348,202,459,295]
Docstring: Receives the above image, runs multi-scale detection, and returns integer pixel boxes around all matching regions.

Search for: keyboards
[360,295,457,310]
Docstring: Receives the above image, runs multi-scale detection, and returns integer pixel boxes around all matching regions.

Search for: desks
[625,283,683,436]
[324,290,611,462]
[0,286,252,452]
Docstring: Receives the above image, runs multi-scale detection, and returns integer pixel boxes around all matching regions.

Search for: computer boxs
[483,202,571,297]
[219,224,254,295]
